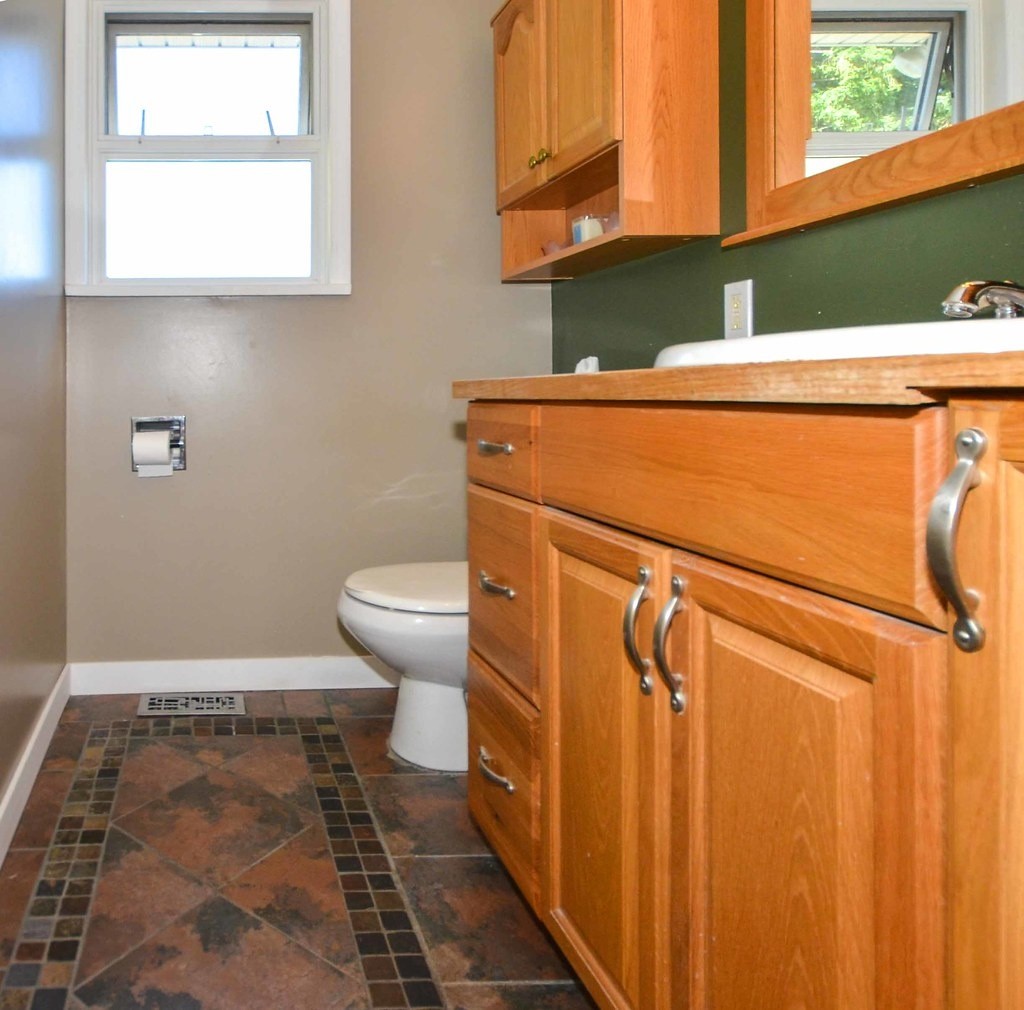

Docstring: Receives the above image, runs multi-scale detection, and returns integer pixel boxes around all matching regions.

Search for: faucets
[941,279,1024,319]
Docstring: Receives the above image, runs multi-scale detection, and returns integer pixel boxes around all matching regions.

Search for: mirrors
[764,0,1024,194]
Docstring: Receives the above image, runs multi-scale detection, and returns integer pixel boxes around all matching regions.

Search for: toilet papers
[132,430,174,478]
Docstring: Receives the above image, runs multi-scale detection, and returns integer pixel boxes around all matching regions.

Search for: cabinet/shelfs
[483,2,723,288]
[457,391,1024,1010]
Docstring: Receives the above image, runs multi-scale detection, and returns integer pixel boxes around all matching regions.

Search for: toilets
[335,560,468,773]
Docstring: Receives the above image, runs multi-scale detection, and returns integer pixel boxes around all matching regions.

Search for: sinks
[652,320,1024,369]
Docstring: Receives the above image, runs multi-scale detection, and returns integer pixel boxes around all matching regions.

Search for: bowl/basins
[571,214,608,246]
